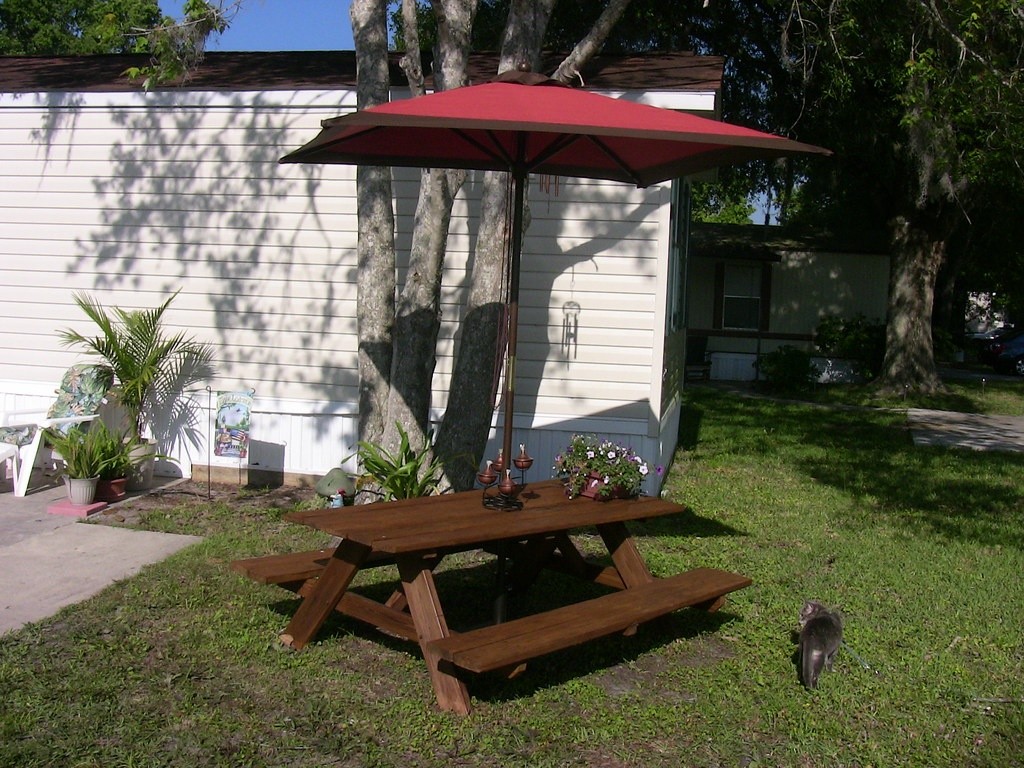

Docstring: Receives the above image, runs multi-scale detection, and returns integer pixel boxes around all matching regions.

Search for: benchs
[231,538,540,650]
[426,564,751,714]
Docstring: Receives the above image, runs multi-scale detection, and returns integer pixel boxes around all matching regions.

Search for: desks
[272,478,689,712]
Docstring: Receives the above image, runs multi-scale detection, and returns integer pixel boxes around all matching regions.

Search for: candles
[519,442,525,450]
[505,467,512,476]
[487,460,493,468]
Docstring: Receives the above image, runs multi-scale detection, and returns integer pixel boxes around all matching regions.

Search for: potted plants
[87,418,182,501]
[55,284,211,489]
[39,421,121,503]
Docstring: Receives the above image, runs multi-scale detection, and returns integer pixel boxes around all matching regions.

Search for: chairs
[0,363,115,496]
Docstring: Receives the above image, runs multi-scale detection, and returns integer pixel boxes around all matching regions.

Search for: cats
[798,601,843,688]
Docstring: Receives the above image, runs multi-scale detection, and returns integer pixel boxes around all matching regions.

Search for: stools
[0,442,19,488]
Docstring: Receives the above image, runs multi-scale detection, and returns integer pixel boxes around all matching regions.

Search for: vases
[568,471,629,500]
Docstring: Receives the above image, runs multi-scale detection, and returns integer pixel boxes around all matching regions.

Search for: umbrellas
[278,62,832,482]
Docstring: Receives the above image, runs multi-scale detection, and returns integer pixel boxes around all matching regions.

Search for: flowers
[550,433,666,500]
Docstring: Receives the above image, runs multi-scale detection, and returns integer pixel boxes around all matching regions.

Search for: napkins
[498,449,503,454]
